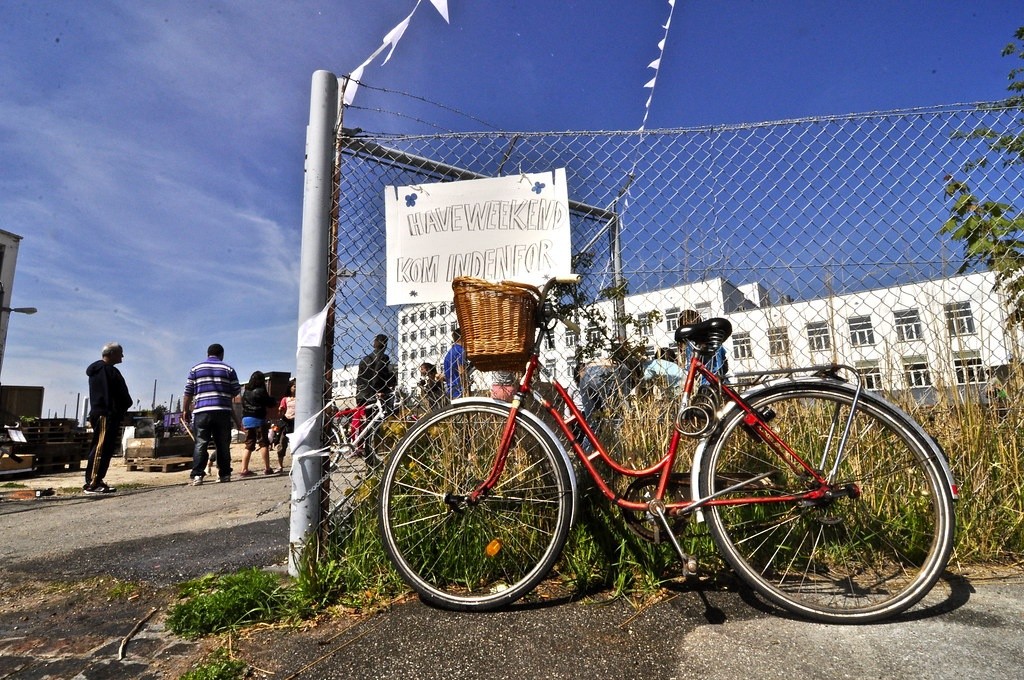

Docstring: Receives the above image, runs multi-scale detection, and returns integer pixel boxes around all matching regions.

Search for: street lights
[0,281,37,328]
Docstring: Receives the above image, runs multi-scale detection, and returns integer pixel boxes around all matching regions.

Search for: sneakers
[85,485,117,494]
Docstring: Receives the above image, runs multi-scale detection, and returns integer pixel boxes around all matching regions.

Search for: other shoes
[265,470,272,474]
[241,471,254,477]
[204,467,212,476]
[274,468,283,473]
[193,476,202,485]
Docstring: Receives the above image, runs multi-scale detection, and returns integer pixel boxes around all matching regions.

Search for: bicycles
[378,274,960,626]
[329,393,390,471]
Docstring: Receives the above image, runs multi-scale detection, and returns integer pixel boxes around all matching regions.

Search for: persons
[490,309,729,455]
[416,363,449,411]
[356,334,397,469]
[183,343,240,485]
[444,328,470,399]
[240,371,277,477]
[82,342,133,495]
[274,379,297,475]
[269,403,402,455]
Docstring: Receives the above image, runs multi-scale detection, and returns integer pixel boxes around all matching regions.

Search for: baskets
[453,276,541,371]
